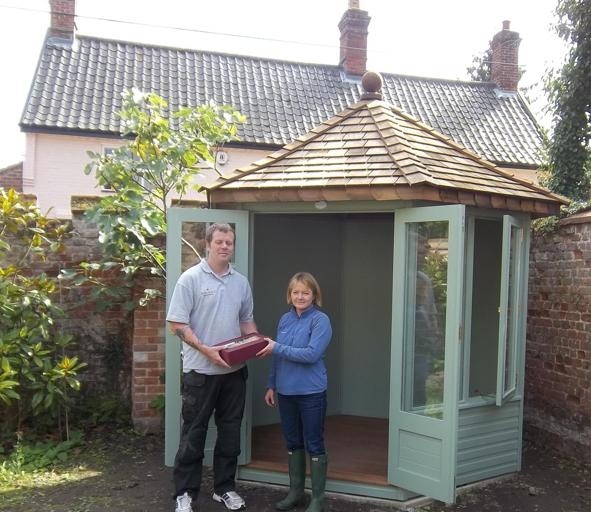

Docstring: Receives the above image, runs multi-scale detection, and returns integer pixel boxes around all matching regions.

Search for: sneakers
[213,491,247,510]
[174,492,194,512]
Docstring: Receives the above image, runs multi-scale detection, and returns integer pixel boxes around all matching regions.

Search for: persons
[166,222,260,512]
[255,271,331,512]
[413,273,442,408]
[417,234,439,336]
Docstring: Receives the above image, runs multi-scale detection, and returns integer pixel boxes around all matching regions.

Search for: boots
[275,448,306,511]
[306,454,328,512]
